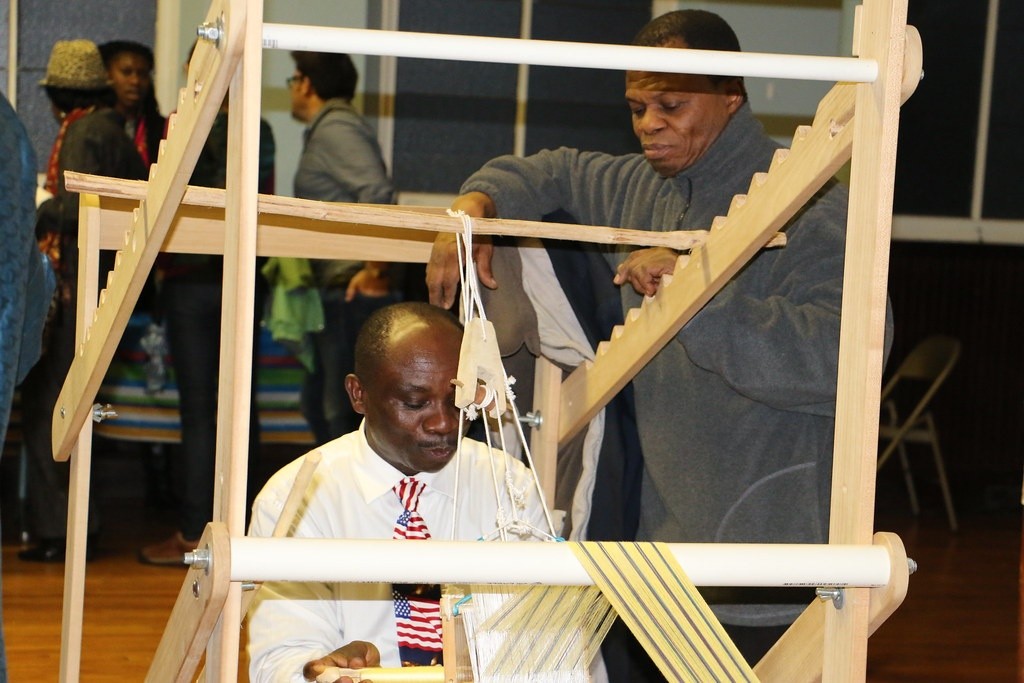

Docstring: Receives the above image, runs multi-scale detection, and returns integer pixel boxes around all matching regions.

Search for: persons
[142,37,276,564]
[18,38,167,563]
[287,50,396,447]
[248,301,610,683]
[424,7,895,683]
[0,91,37,683]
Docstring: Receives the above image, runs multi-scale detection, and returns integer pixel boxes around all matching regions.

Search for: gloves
[35,201,48,242]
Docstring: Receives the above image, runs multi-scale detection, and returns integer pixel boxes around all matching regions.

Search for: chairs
[877,338,959,536]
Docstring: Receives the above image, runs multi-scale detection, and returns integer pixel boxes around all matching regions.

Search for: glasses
[286,74,307,88]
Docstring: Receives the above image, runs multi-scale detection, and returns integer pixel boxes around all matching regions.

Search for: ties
[393,477,443,665]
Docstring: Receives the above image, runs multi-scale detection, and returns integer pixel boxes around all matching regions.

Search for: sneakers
[138,532,200,567]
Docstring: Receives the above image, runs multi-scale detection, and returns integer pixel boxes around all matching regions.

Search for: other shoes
[16,536,108,565]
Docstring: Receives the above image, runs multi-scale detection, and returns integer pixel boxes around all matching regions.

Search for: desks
[15,332,312,558]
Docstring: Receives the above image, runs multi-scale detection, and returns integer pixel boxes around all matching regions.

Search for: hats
[37,40,116,88]
